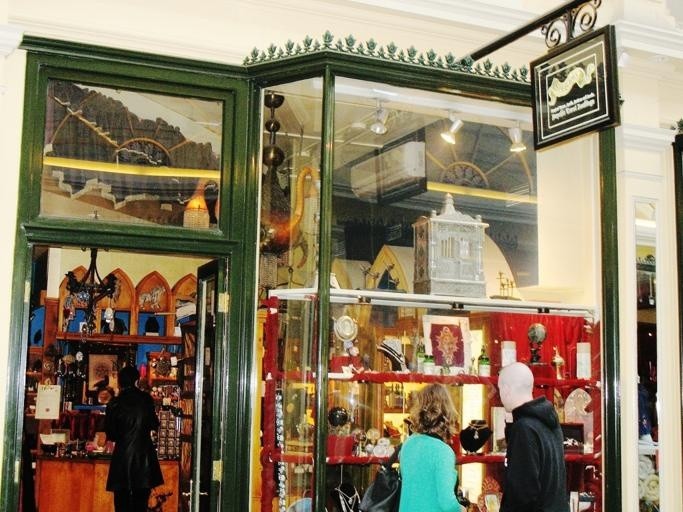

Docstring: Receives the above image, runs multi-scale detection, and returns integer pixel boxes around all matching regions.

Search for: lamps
[508,119,526,153]
[440,112,464,144]
[370,97,387,135]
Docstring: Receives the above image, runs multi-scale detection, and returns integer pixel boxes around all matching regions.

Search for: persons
[398,383,467,512]
[498,362,570,512]
[104,366,165,512]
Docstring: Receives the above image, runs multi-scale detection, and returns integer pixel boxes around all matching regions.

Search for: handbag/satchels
[357,442,404,511]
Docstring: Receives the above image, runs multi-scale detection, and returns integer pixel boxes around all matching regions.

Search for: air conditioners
[349,136,426,207]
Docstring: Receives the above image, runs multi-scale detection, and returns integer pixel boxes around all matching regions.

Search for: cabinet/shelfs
[262,304,604,512]
[25,280,202,511]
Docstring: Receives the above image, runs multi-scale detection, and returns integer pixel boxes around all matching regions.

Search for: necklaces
[469,424,488,439]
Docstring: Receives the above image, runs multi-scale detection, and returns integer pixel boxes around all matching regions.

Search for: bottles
[415,341,435,374]
[478,344,491,377]
